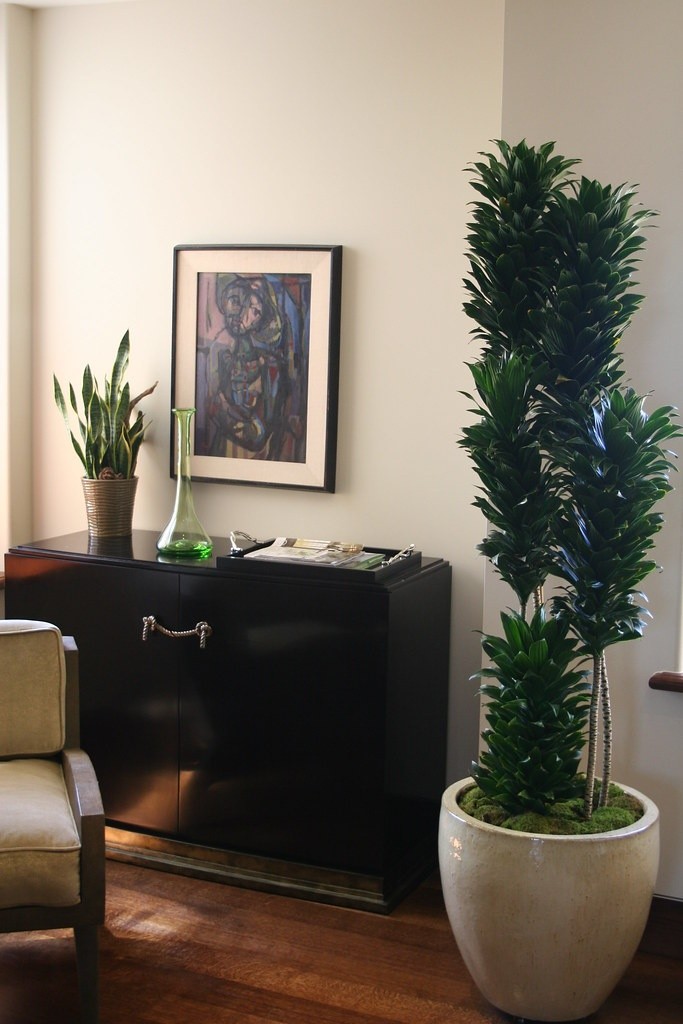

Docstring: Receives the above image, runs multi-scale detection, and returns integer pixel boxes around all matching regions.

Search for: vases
[157,408,213,557]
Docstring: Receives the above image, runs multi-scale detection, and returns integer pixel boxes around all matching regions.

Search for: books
[244,536,385,570]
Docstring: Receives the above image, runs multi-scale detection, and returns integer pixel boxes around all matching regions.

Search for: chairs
[0,619,108,1024]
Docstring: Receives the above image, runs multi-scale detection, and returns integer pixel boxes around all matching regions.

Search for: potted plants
[440,138,683,1024]
[53,330,156,539]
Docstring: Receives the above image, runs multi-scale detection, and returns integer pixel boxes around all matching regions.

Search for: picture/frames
[168,245,343,494]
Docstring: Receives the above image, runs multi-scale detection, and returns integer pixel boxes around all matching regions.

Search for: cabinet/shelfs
[5,527,452,915]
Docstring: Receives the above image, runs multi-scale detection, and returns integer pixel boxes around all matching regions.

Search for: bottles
[153,409,215,561]
